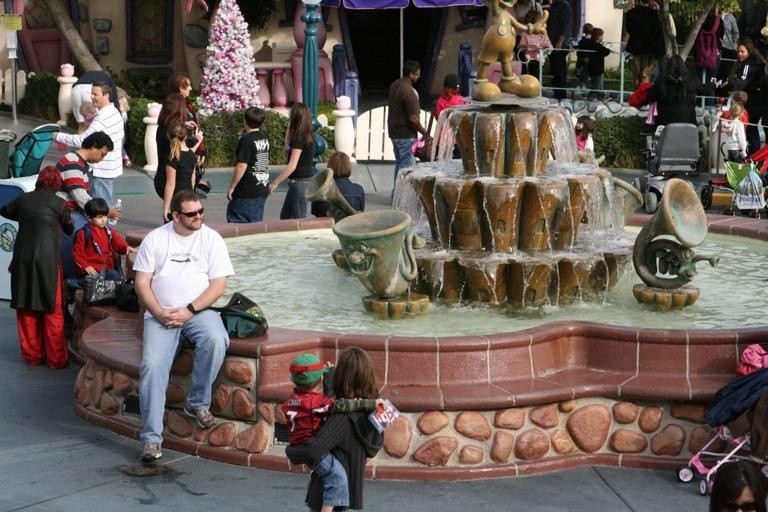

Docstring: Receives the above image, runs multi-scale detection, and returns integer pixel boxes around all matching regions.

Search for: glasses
[177,208,206,218]
[725,499,753,512]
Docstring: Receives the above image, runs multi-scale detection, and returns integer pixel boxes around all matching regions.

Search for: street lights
[288,1,326,189]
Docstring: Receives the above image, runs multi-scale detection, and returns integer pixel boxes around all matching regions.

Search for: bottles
[107,199,121,226]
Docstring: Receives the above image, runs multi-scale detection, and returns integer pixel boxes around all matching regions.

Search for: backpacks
[694,17,721,73]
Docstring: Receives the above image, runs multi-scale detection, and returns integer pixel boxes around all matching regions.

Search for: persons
[50,70,131,206]
[513,1,611,101]
[286,344,381,512]
[0,132,139,368]
[623,1,768,169]
[131,184,235,459]
[575,115,594,152]
[310,150,366,220]
[387,58,431,201]
[708,459,766,512]
[434,73,473,161]
[153,71,210,201]
[266,101,315,219]
[224,104,270,223]
[281,354,389,512]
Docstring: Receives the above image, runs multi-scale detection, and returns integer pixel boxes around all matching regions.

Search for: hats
[290,354,330,385]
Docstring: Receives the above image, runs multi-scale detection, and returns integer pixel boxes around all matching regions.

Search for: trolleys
[8,123,62,180]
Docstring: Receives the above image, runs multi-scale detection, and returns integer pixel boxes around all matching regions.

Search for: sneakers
[140,440,161,462]
[183,405,217,428]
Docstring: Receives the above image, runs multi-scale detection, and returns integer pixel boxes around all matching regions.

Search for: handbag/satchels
[220,292,269,338]
[85,277,140,311]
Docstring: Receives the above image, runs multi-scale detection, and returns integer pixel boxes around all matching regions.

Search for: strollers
[675,343,768,497]
[720,141,768,219]
[633,123,703,213]
